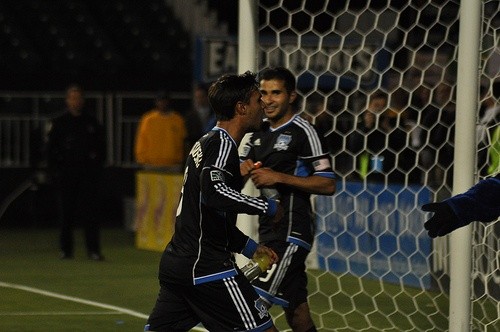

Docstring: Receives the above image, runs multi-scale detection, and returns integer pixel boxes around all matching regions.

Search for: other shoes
[88,252,106,260]
[57,248,74,260]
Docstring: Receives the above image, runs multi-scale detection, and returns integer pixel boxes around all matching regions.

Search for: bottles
[241,253,274,281]
[262,187,279,199]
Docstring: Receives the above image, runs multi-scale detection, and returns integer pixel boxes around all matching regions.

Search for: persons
[143,71,284,332]
[421,173,499,239]
[236,65,336,332]
[294,73,500,187]
[131,82,217,172]
[38,85,111,261]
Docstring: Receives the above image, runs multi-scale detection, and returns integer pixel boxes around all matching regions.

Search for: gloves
[421,194,479,238]
